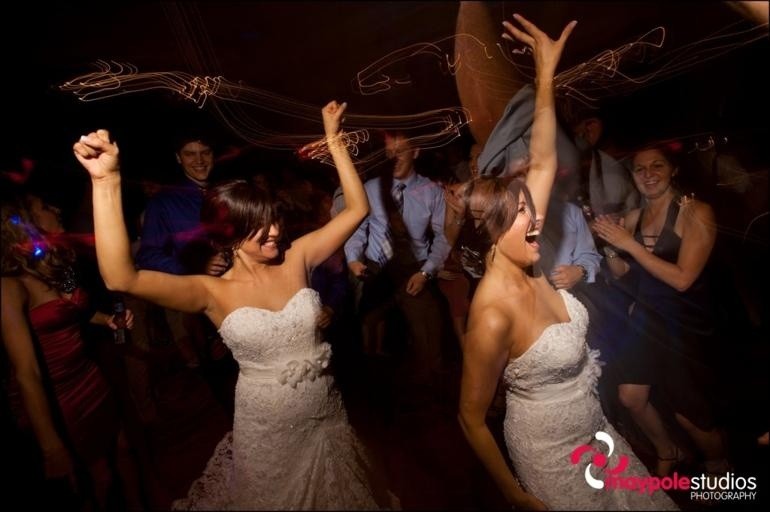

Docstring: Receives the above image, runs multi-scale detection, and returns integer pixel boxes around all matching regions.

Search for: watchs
[576,264,589,283]
[419,270,432,281]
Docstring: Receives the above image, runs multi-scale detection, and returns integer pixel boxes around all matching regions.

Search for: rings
[521,45,528,53]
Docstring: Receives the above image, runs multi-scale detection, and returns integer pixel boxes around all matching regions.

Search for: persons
[134,125,240,275]
[461,154,602,297]
[329,126,454,429]
[0,189,136,512]
[456,14,682,512]
[442,143,506,419]
[72,100,402,512]
[592,141,735,508]
[193,166,347,331]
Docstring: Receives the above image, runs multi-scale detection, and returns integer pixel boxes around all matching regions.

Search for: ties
[377,182,407,271]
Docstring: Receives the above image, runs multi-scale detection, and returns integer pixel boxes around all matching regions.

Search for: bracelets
[453,216,467,226]
[603,246,625,259]
